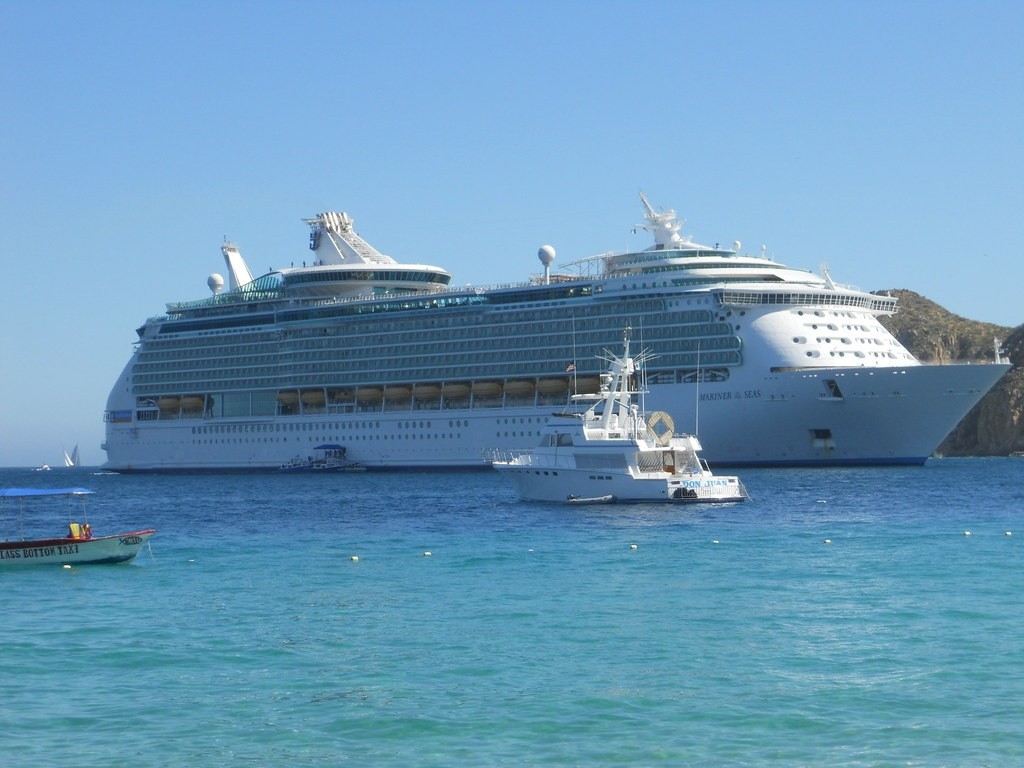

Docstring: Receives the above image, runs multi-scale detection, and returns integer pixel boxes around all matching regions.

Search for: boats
[482,318,753,504]
[32,464,52,471]
[280,444,367,473]
[90,471,120,476]
[0,488,156,566]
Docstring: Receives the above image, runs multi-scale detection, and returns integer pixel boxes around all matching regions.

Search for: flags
[562,360,574,372]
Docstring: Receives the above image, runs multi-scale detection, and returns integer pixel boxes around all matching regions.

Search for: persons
[308,456,312,463]
[329,449,345,458]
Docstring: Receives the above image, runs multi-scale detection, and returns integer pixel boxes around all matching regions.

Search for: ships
[99,192,1012,471]
